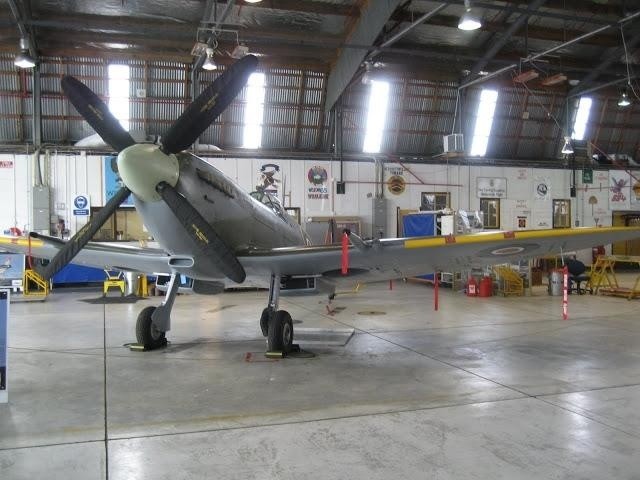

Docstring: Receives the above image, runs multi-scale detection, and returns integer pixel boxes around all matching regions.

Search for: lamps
[560,135,575,154]
[201,44,218,70]
[13,37,36,68]
[617,84,630,107]
[230,47,249,59]
[456,0,482,30]
[361,62,376,85]
[192,41,209,57]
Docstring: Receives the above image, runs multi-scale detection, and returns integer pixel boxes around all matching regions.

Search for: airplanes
[0,53,639,357]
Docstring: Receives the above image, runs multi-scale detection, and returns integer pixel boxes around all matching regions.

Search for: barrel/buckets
[478,277,491,297]
[468,278,477,295]
[122,271,140,298]
[549,268,564,296]
